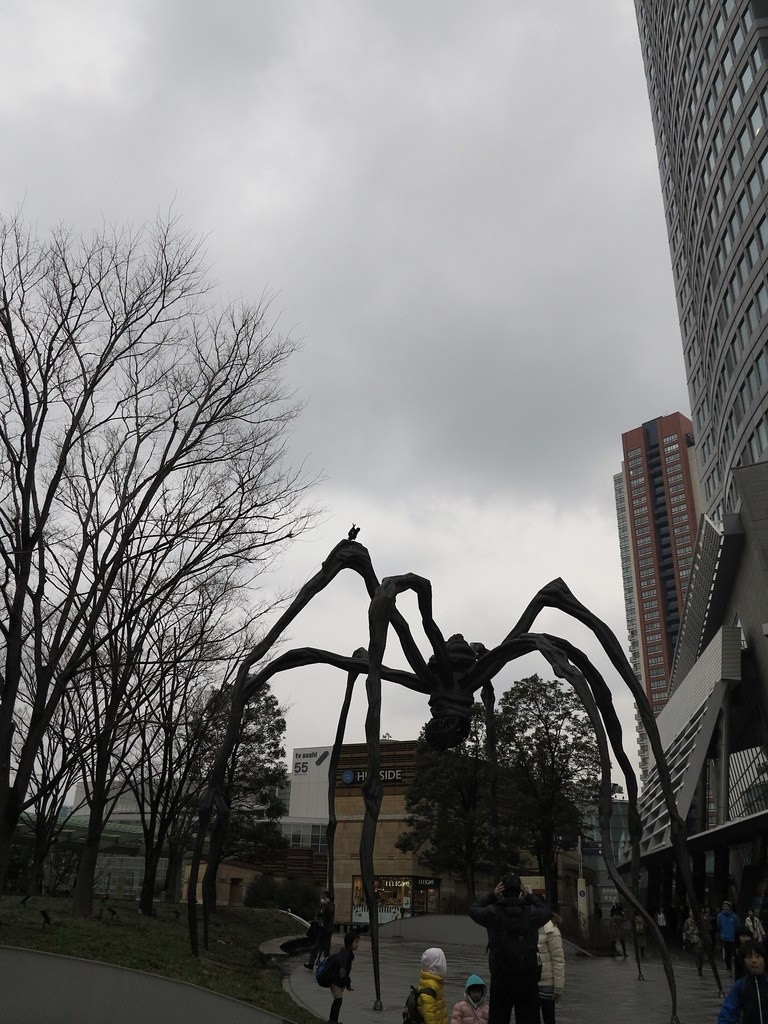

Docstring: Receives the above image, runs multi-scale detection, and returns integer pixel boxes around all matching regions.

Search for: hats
[501,873,521,897]
[724,901,732,905]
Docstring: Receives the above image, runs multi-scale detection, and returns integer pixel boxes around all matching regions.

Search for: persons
[610,902,628,958]
[718,940,768,1024]
[417,947,449,1024]
[735,926,754,983]
[745,907,766,943]
[635,910,649,960]
[689,904,720,954]
[531,893,565,1024]
[717,900,741,978]
[451,973,489,1024]
[304,891,335,970]
[469,869,553,1024]
[327,930,360,1024]
[594,902,602,922]
[654,904,667,932]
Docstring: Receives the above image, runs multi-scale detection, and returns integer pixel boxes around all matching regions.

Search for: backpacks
[402,984,436,1024]
[315,953,351,987]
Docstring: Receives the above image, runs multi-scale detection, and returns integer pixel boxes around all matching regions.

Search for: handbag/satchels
[306,921,319,938]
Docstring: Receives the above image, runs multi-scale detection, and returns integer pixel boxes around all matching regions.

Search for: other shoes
[303,962,315,970]
